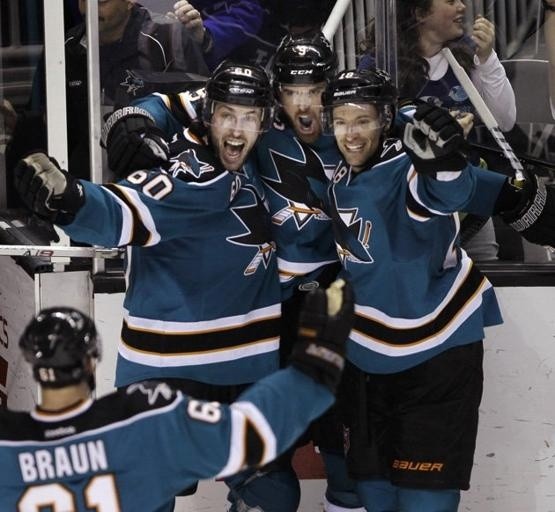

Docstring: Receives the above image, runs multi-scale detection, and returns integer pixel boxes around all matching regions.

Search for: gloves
[99,107,171,175]
[402,105,466,174]
[15,154,84,226]
[288,278,354,387]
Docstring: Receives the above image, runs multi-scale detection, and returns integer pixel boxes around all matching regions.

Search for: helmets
[203,34,398,129]
[19,308,101,388]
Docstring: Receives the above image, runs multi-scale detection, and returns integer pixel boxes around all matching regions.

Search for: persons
[0,279,356,512]
[100,32,555,512]
[11,58,301,512]
[320,70,504,512]
